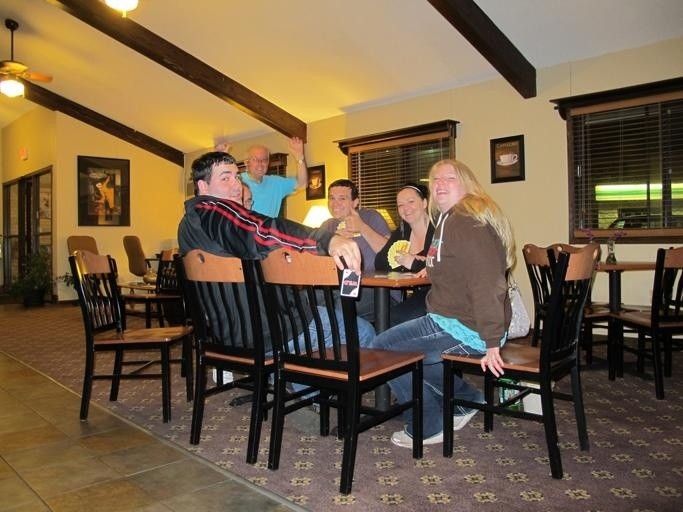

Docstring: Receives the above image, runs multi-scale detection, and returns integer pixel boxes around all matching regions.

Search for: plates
[496,160,517,166]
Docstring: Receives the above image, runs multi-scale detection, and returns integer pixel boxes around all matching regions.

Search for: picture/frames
[77,154,131,227]
[305,164,326,200]
[490,133,525,184]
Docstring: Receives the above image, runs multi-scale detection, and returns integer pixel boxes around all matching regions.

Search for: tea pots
[142,259,156,284]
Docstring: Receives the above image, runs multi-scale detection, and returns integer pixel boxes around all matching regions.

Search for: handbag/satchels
[506,272,532,339]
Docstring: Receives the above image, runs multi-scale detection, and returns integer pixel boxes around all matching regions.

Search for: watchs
[297,159,305,165]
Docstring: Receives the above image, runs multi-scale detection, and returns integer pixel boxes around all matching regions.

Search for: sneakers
[212,366,234,386]
[390,429,443,448]
[452,399,487,430]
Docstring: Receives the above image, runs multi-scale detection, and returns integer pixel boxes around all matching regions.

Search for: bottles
[606,252,616,265]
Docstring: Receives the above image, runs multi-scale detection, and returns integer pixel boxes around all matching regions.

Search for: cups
[129,281,144,287]
[500,154,517,163]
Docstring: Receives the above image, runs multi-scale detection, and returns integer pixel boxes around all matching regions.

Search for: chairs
[440,240,601,479]
[523,244,681,400]
[172,247,432,495]
[66,235,196,424]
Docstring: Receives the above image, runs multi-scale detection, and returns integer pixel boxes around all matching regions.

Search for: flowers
[606,228,627,241]
[578,225,596,241]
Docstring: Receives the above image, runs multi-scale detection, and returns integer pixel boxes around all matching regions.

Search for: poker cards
[386,240,410,270]
[340,269,360,298]
[336,221,360,238]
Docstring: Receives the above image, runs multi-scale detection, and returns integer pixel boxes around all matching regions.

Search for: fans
[0,19,54,83]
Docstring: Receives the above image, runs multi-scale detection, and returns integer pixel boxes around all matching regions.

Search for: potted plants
[8,238,76,310]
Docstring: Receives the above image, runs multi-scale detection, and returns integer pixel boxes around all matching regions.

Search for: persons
[178,152,376,413]
[242,182,254,211]
[216,136,308,219]
[374,183,439,328]
[366,158,531,451]
[320,179,403,323]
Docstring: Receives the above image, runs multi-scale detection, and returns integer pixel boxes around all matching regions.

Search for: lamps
[0,74,25,98]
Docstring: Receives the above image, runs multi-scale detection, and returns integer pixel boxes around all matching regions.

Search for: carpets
[0,305,682,511]
